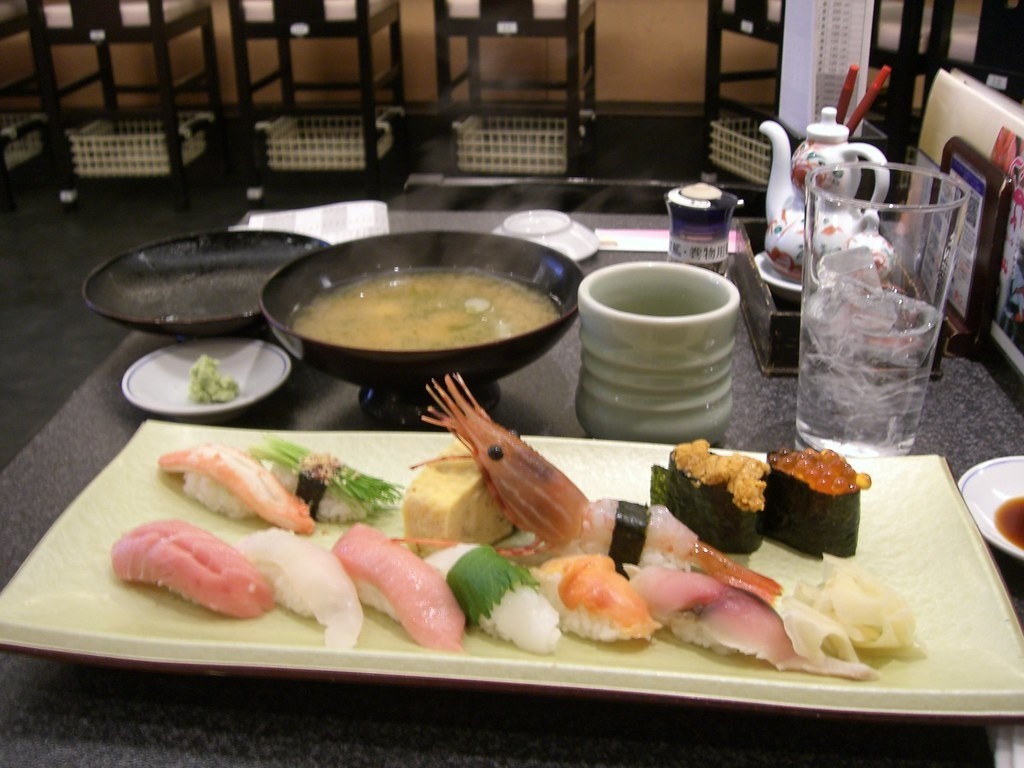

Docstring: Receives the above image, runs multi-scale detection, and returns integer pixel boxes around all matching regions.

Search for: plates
[119,338,290,414]
[1,418,1024,723]
[752,251,805,295]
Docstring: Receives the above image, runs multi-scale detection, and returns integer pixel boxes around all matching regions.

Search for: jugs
[758,106,889,284]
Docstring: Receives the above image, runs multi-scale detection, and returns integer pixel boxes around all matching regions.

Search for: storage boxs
[727,218,950,383]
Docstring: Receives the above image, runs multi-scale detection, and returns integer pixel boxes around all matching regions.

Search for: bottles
[661,182,741,280]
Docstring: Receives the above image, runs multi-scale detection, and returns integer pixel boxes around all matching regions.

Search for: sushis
[111,370,881,682]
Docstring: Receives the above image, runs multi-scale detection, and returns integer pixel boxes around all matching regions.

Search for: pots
[258,229,582,423]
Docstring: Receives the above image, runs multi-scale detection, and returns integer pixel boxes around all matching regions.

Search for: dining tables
[0,197,1024,768]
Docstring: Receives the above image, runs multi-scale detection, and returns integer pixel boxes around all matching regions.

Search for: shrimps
[419,372,784,603]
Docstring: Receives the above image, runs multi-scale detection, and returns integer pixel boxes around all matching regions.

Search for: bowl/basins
[80,230,332,339]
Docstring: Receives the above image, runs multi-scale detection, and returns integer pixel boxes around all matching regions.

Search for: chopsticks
[835,66,891,137]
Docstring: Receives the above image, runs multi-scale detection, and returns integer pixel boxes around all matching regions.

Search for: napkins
[226,200,391,248]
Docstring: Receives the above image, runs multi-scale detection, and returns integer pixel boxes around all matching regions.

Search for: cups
[793,163,965,457]
[575,260,740,445]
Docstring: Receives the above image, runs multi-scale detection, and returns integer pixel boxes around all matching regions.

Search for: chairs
[0,0,1024,200]
